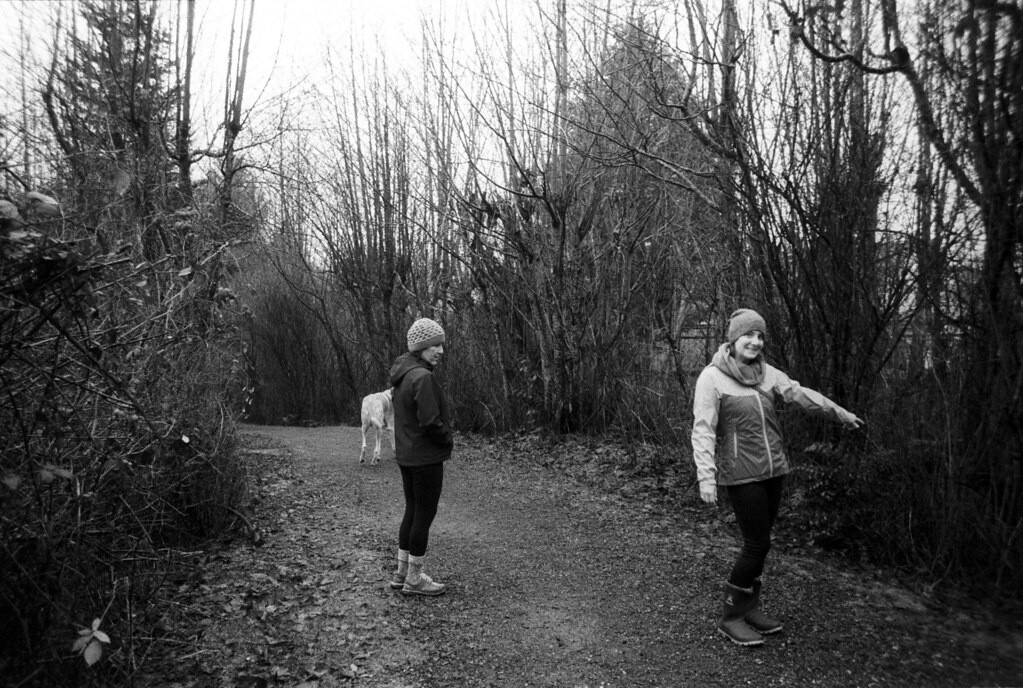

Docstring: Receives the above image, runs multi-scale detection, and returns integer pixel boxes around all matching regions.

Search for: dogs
[359,388,396,466]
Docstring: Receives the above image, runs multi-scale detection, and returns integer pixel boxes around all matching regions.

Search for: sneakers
[390,572,433,589]
[402,573,446,596]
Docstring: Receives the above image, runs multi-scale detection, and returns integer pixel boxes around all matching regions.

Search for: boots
[717,579,765,645]
[745,579,783,634]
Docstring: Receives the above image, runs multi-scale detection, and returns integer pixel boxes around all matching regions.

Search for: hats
[406,318,445,352]
[728,309,767,346]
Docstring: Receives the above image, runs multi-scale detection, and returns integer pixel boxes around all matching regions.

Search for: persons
[389,317,455,595]
[691,309,865,646]
